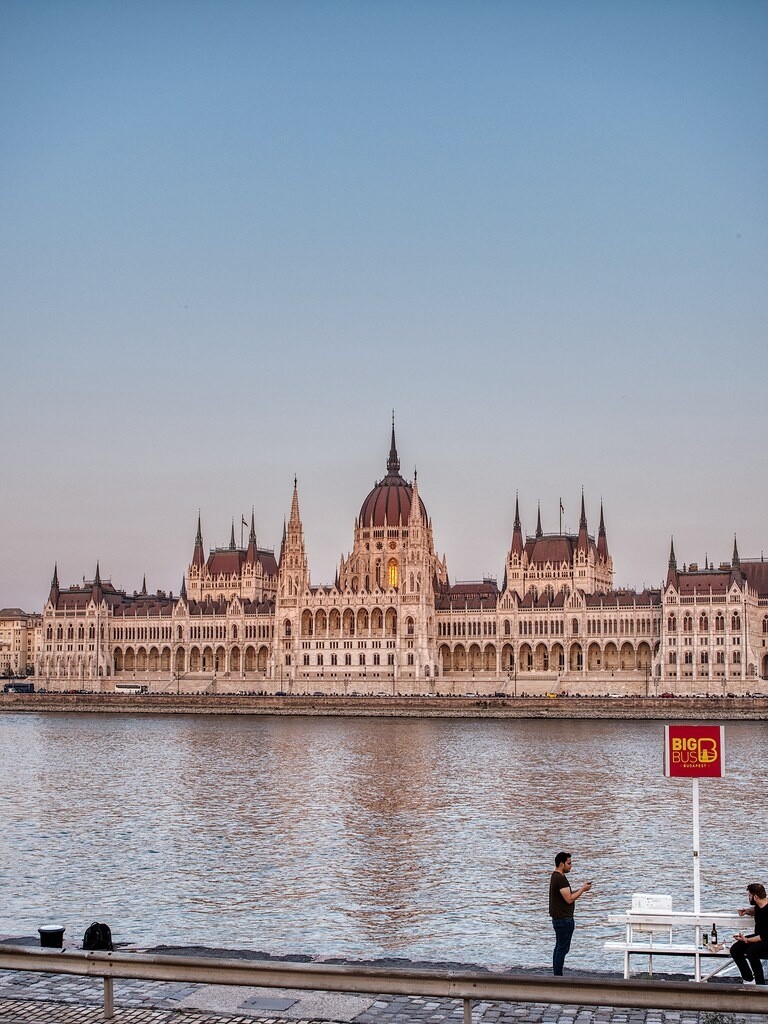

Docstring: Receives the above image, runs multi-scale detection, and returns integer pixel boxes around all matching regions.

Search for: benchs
[604,913,755,984]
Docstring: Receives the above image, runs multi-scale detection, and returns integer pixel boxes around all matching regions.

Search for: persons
[549,852,592,975]
[730,884,768,985]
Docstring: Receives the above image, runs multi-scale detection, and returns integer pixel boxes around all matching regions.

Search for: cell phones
[733,934,741,937]
[588,881,593,885]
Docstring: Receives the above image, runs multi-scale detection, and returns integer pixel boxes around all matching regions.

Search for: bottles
[711,923,717,944]
[703,930,708,946]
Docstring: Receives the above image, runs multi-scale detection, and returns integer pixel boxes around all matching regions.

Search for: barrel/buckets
[38,925,66,948]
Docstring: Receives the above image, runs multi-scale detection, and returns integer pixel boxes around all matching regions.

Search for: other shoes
[743,978,756,984]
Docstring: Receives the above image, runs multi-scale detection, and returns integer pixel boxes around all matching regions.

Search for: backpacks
[83,921,113,950]
